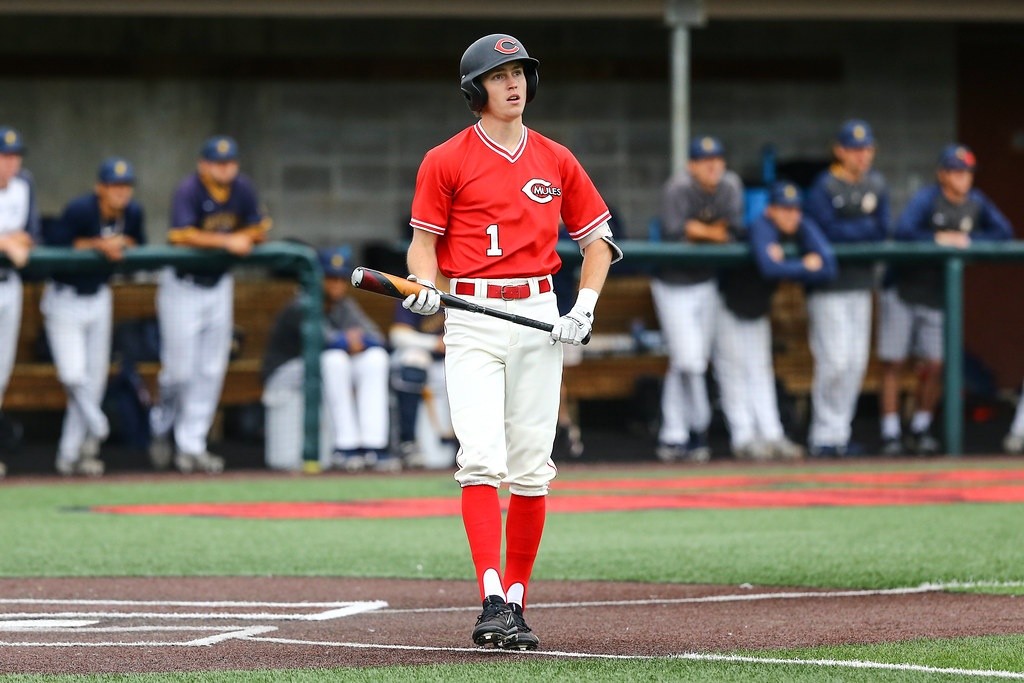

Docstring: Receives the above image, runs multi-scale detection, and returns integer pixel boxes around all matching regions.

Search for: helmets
[459,34,540,110]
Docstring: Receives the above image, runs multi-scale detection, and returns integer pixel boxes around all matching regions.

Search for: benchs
[0,271,926,453]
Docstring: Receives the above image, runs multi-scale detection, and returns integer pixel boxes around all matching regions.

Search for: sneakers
[494,603,539,651]
[472,595,519,648]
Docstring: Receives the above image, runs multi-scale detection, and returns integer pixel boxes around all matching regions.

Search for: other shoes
[332,449,367,472]
[173,450,224,474]
[655,430,711,464]
[366,448,404,473]
[149,432,173,467]
[881,437,901,456]
[54,430,106,477]
[903,429,939,454]
[729,437,772,462]
[771,436,805,459]
[810,447,845,457]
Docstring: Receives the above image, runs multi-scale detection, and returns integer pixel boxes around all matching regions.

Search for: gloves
[402,275,441,315]
[549,306,594,347]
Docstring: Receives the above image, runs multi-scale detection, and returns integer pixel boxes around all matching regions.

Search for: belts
[455,279,551,302]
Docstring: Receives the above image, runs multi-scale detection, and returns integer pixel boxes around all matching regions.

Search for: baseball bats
[351,266,591,345]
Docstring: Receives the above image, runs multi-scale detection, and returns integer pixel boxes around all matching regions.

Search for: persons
[400,34,624,651]
[794,118,892,458]
[39,157,150,477]
[260,242,583,468]
[649,135,745,461]
[146,137,275,473]
[1003,390,1024,455]
[0,124,40,478]
[876,144,1015,455]
[711,181,837,458]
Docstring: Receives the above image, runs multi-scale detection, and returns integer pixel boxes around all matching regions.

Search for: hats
[320,246,354,275]
[770,181,804,208]
[202,138,239,161]
[940,145,976,170]
[0,128,25,155]
[98,159,137,184]
[838,119,876,151]
[688,135,724,159]
